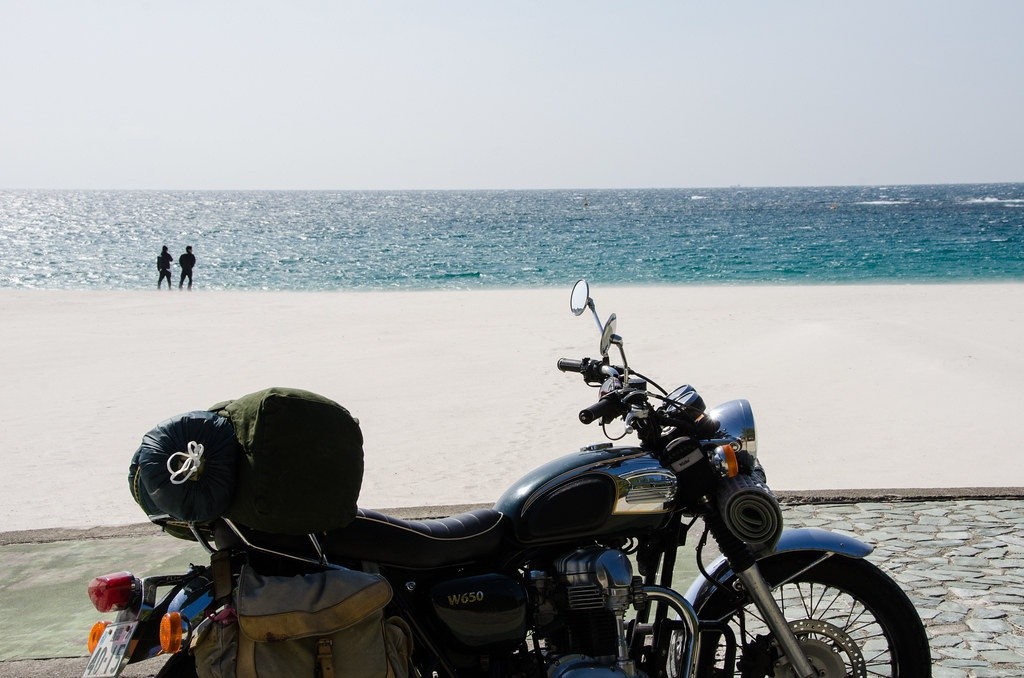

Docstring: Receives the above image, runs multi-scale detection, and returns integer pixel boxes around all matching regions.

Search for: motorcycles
[82,277,932,678]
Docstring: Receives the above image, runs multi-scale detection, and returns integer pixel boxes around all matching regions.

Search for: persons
[177,246,196,289]
[155,245,174,290]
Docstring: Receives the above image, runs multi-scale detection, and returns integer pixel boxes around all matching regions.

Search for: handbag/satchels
[190,563,414,678]
